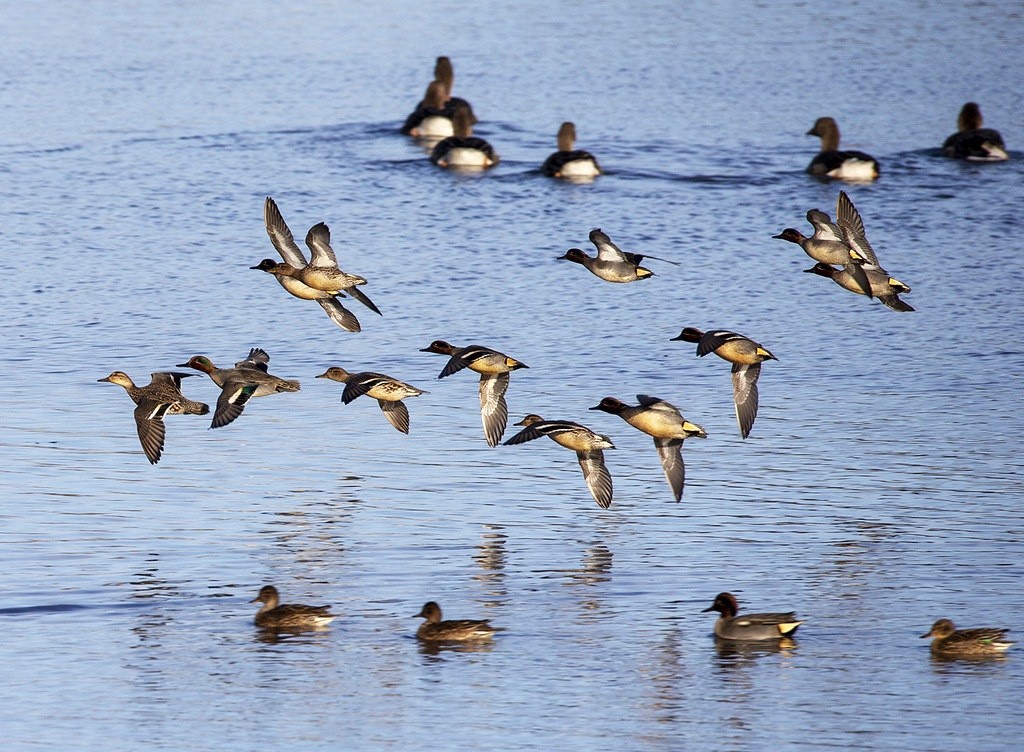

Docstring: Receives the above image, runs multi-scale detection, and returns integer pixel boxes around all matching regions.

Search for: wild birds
[316,366,430,434]
[411,602,509,645]
[247,584,337,629]
[504,415,620,510]
[250,199,382,333]
[668,326,782,440]
[398,55,503,169]
[588,393,710,504]
[939,102,1010,164]
[919,618,1020,657]
[805,116,883,184]
[97,371,211,465]
[557,228,681,285]
[702,591,807,644]
[420,340,530,448]
[175,346,302,431]
[540,121,601,179]
[770,190,917,314]
